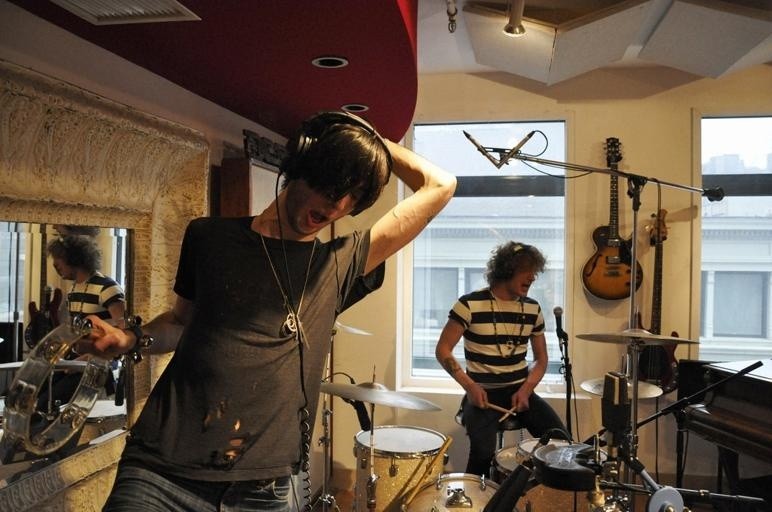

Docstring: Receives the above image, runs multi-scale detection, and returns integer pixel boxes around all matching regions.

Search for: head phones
[282,111,395,183]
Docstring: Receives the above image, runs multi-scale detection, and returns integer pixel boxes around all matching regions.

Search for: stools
[454,408,530,449]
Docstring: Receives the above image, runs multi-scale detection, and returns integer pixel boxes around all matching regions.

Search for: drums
[353,426,447,511]
[400,472,520,512]
[533,443,611,491]
[516,439,587,472]
[490,446,605,511]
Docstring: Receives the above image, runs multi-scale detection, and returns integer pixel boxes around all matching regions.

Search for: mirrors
[0,196,153,511]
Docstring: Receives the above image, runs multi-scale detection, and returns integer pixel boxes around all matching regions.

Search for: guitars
[624,210,680,396]
[581,138,642,300]
[24,223,63,350]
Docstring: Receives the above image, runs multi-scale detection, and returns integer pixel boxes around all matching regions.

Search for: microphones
[554,307,567,342]
[350,378,370,434]
[478,431,551,511]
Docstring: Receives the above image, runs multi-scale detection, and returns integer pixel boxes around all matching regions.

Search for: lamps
[502,1,527,38]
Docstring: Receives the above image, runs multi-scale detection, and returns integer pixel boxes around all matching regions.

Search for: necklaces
[69,280,90,324]
[258,212,321,354]
[487,291,525,361]
[492,295,521,349]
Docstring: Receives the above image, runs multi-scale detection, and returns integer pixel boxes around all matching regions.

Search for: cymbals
[320,382,442,411]
[577,328,698,345]
[581,378,663,399]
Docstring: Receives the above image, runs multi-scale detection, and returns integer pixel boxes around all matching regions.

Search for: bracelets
[113,313,155,368]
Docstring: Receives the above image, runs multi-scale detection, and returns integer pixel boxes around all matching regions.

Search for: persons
[434,240,574,479]
[69,109,461,511]
[48,224,104,239]
[36,236,128,415]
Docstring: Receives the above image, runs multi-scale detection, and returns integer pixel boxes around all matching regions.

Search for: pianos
[676,359,771,512]
